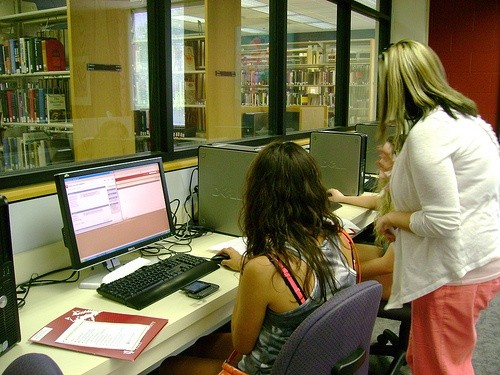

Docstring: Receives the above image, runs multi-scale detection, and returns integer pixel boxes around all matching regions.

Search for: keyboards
[97,252,219,310]
[364,175,379,192]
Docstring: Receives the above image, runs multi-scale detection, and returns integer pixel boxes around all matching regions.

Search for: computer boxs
[241,111,264,138]
[287,111,299,131]
[355,123,399,174]
[0,194,20,358]
[197,142,263,236]
[310,130,367,196]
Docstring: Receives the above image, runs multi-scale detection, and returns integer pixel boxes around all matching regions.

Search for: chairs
[273,278,384,375]
[370,302,412,375]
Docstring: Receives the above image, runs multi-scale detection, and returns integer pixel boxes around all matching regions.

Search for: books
[134,40,206,152]
[327,53,369,116]
[241,68,325,106]
[0,36,73,172]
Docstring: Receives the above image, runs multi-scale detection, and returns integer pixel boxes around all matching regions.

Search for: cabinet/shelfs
[0,0,137,173]
[323,38,374,123]
[242,40,320,63]
[137,0,243,155]
[241,62,327,133]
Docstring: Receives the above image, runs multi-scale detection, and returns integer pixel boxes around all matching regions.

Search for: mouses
[210,253,230,263]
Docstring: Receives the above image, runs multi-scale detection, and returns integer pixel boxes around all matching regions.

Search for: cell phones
[181,280,210,293]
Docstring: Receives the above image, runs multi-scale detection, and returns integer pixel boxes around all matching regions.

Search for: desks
[0,193,378,375]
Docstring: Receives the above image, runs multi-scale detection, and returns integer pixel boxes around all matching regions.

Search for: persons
[375,40,500,374]
[328,134,409,300]
[173,142,361,375]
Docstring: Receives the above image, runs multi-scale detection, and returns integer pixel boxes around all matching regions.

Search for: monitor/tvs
[55,156,176,290]
